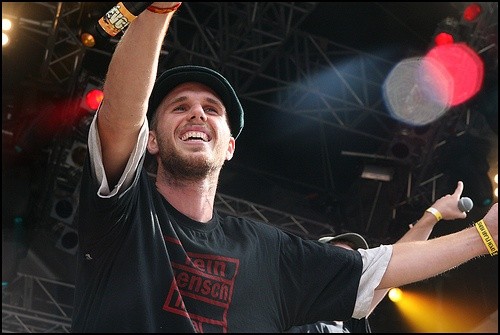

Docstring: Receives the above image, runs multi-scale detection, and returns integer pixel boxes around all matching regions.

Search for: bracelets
[475,219,498,256]
[147,2,181,14]
[426,207,443,221]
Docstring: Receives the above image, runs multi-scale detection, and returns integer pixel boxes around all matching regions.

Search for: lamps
[81,8,105,47]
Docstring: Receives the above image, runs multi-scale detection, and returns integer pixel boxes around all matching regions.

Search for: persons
[70,2,498,333]
[284,181,466,333]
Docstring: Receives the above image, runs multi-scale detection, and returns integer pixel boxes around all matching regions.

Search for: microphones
[409,197,473,229]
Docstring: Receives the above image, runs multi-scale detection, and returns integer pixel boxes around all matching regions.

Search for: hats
[146,65,243,140]
[318,233,369,250]
